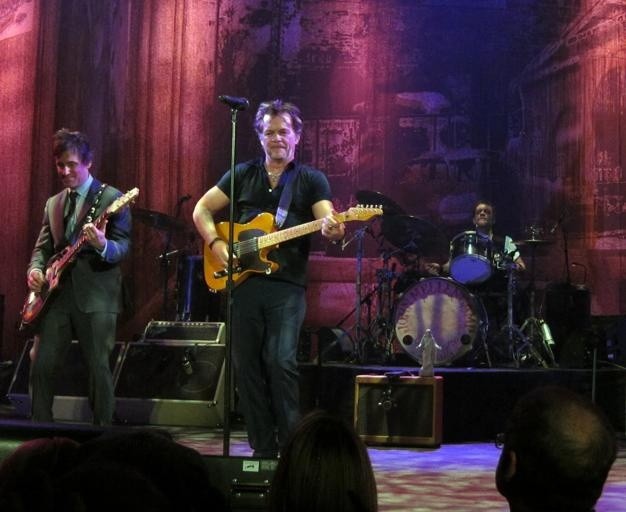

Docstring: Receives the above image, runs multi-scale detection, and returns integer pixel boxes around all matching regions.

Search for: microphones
[158,248,186,259]
[550,211,566,234]
[216,95,250,112]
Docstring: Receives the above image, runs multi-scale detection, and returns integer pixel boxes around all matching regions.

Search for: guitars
[202,204,382,294]
[18,187,141,328]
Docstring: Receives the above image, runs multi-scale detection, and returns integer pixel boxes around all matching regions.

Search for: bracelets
[208,237,223,250]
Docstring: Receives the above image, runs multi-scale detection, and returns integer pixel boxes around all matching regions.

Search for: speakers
[111,340,234,428]
[6,338,126,424]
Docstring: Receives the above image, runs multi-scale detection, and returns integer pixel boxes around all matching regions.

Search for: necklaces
[266,170,283,179]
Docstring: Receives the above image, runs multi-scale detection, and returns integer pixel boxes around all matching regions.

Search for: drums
[392,277,489,365]
[449,231,496,286]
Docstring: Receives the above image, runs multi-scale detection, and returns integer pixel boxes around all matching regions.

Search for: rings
[328,226,334,230]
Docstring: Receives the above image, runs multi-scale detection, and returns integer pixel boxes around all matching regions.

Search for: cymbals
[355,189,406,218]
[133,206,196,244]
[520,237,554,243]
[378,213,450,257]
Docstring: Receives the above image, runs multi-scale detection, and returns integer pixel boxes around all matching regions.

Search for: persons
[191,98,346,456]
[267,407,379,510]
[493,382,620,510]
[24,126,132,430]
[1,426,233,510]
[423,200,524,353]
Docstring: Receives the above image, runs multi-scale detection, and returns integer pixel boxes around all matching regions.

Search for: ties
[63,192,77,235]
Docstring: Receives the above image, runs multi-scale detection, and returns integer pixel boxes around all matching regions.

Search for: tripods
[480,324,552,370]
